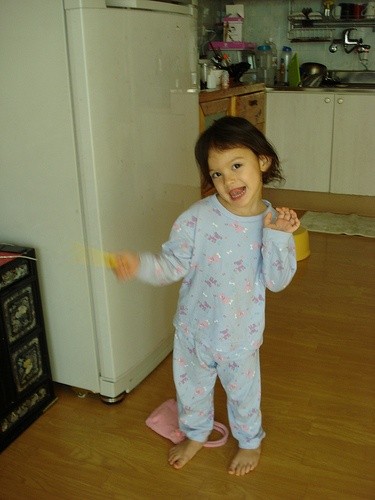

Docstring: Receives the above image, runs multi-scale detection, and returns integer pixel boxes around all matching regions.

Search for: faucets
[329,29,372,55]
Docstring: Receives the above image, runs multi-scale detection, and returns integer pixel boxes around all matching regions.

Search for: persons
[110,116,300,477]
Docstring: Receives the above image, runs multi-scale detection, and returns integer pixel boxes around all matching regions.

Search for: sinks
[330,67,375,89]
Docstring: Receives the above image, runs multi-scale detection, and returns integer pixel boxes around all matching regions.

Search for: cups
[257,45,272,85]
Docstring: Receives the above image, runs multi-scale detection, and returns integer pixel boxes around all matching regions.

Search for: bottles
[206,69,216,89]
[222,70,230,87]
[281,47,290,85]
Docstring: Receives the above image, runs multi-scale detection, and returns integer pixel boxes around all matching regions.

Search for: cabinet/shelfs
[0,244,58,456]
[199,81,375,218]
[287,0,375,46]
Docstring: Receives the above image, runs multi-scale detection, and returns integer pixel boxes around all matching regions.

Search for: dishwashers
[264,92,334,194]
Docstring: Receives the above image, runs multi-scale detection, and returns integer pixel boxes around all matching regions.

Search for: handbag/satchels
[147,398,228,449]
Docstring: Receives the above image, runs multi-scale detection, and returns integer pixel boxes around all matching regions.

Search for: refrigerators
[0,0,200,403]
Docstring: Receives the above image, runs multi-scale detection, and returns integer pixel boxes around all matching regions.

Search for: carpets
[299,211,375,240]
